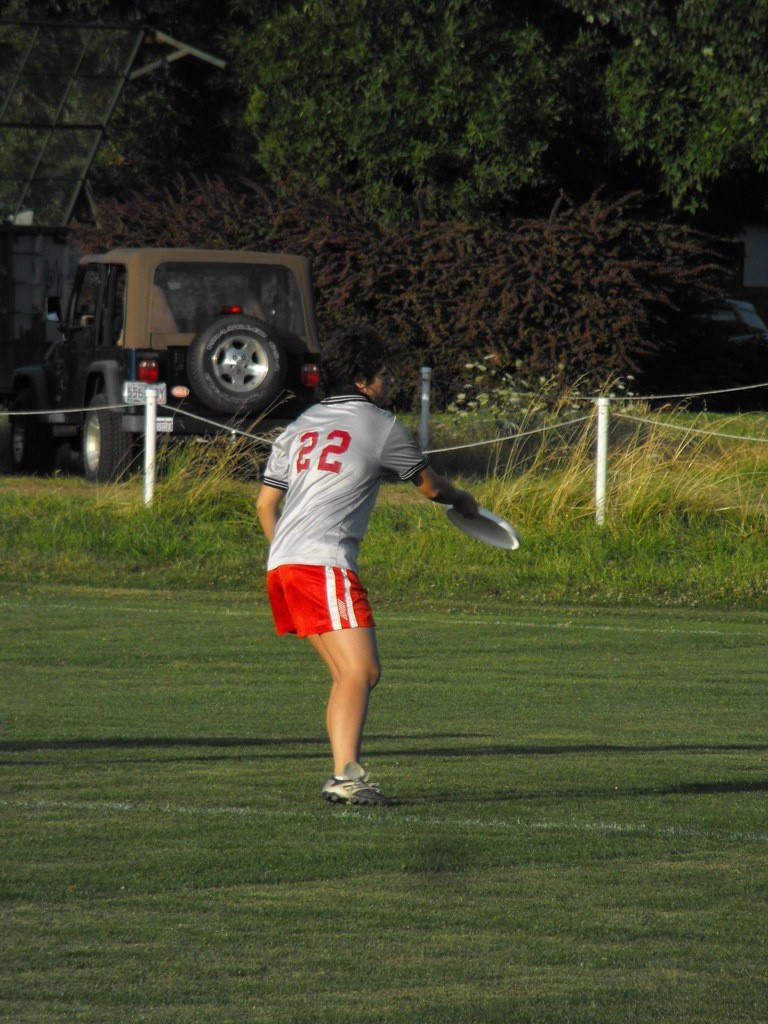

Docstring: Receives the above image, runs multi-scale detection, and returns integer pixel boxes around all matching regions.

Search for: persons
[256,331,481,807]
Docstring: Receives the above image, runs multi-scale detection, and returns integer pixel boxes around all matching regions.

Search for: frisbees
[445,504,521,551]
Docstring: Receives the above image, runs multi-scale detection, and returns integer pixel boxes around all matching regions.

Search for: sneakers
[320,761,401,806]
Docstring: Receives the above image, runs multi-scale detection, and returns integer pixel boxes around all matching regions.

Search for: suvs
[2,246,322,485]
